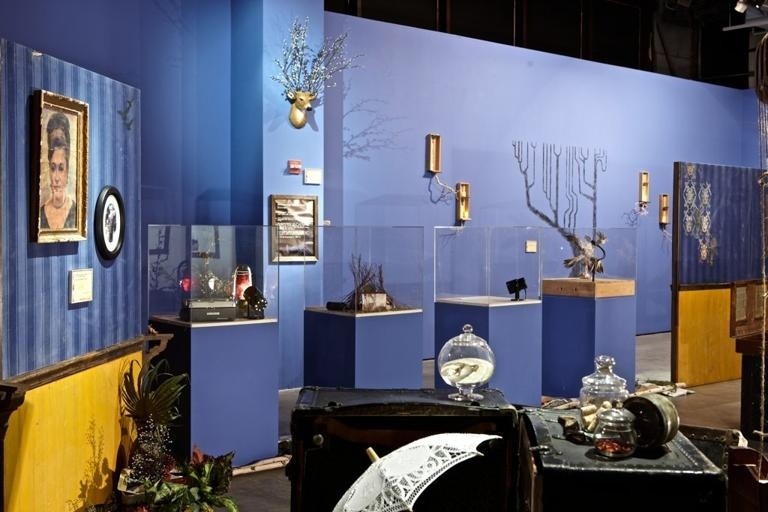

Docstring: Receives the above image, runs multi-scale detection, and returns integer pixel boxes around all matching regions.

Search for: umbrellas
[330,428,504,511]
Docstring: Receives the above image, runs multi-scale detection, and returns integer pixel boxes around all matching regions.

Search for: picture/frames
[95,184,125,260]
[68,268,94,305]
[270,195,319,263]
[29,89,90,245]
[148,225,221,258]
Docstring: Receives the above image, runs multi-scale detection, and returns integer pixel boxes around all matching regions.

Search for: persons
[39,110,75,228]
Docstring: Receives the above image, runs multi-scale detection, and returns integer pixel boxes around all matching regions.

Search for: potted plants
[121,359,189,469]
